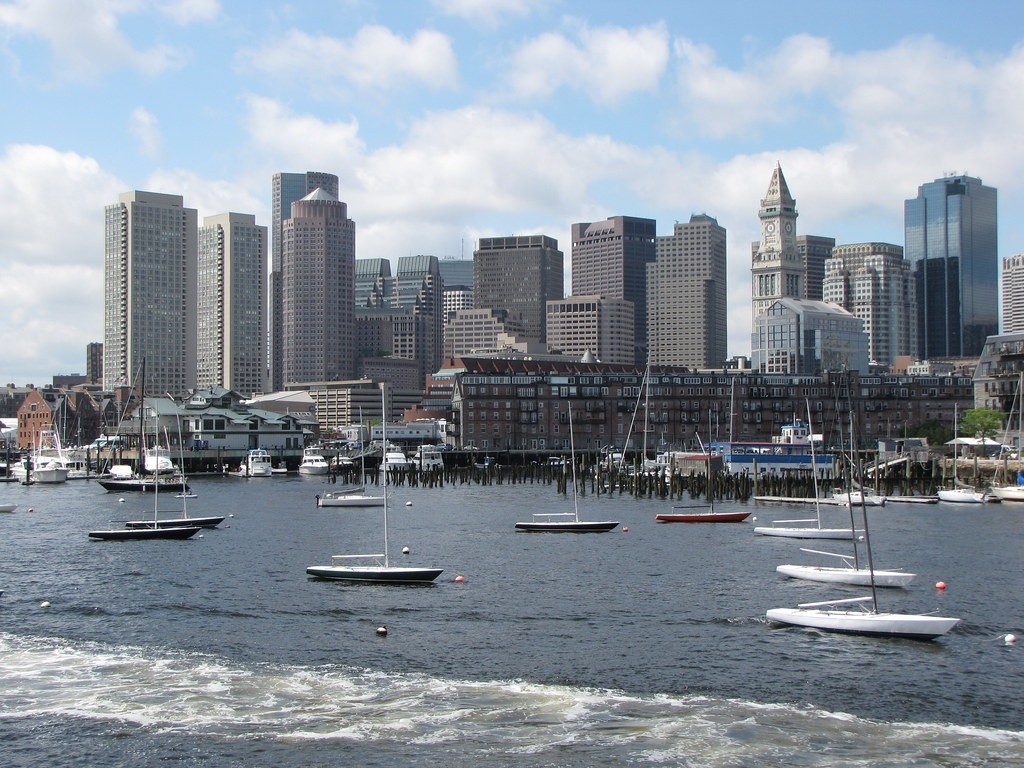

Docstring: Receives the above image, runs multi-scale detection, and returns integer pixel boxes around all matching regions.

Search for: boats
[272,469,288,472]
[412,452,444,471]
[305,384,445,583]
[30,421,70,483]
[329,453,354,473]
[378,453,411,471]
[174,495,198,498]
[228,448,273,477]
[298,446,329,475]
[0,504,17,512]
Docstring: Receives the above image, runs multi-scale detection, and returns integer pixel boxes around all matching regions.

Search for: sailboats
[833,366,887,507]
[764,409,961,642]
[515,401,620,533]
[314,406,385,508]
[990,371,1024,502]
[753,398,866,539]
[938,402,1003,504]
[774,383,917,588]
[654,410,752,522]
[89,356,225,540]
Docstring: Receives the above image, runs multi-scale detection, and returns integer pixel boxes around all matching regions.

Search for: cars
[344,440,479,450]
